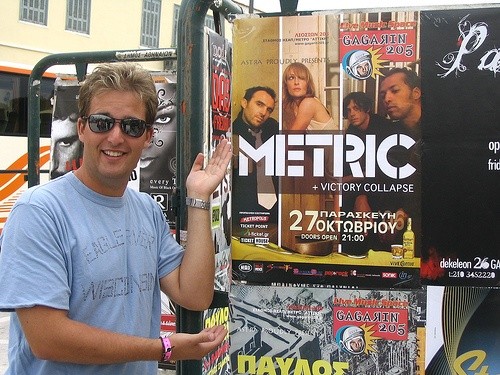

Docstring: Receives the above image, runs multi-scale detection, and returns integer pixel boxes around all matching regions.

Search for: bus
[0,60,177,242]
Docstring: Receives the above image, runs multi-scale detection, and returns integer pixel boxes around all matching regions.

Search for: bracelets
[159,336,174,362]
[186,197,210,210]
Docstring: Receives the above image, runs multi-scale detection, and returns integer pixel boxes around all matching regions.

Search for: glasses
[82,114,150,138]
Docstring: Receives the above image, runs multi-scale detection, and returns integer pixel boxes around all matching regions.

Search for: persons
[140,83,179,193]
[343,68,422,256]
[48,85,84,180]
[232,84,278,225]
[338,326,365,352]
[0,62,234,375]
[282,62,338,187]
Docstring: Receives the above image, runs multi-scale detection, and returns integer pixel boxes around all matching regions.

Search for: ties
[248,128,277,210]
